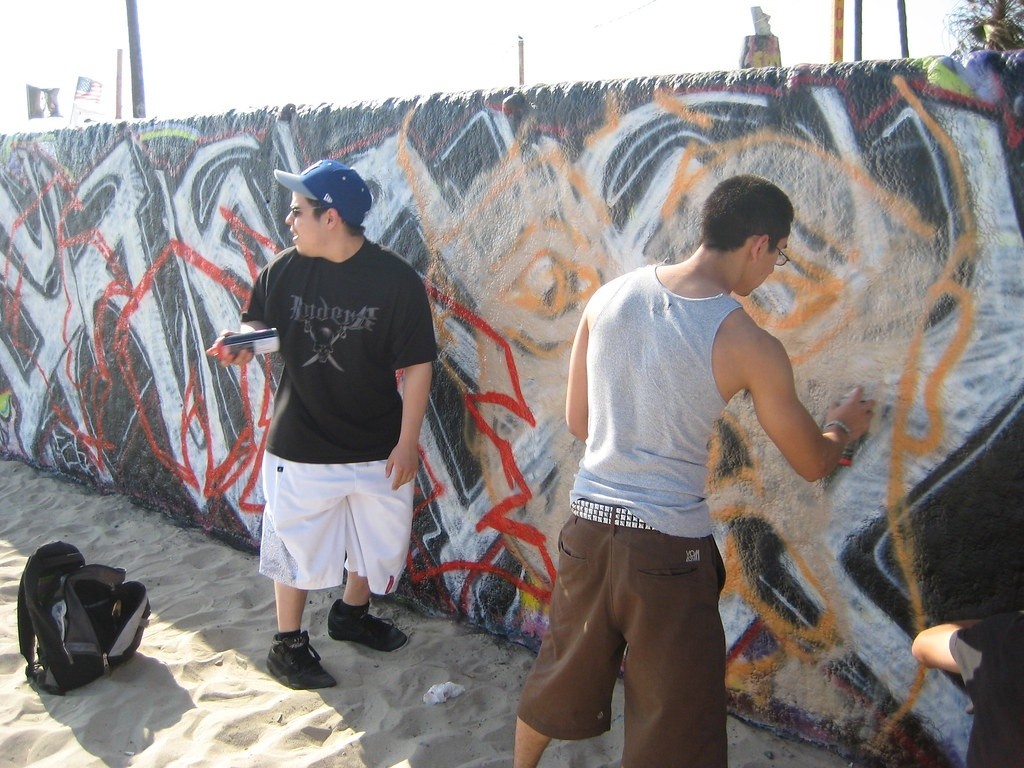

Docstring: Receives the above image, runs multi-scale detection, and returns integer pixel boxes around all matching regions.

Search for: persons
[911,607,1024,768]
[513,172,878,767]
[208,159,439,691]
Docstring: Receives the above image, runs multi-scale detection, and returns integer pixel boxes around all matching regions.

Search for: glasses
[767,237,790,266]
[289,205,330,217]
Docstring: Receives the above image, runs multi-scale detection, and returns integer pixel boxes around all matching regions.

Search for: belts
[570,500,659,531]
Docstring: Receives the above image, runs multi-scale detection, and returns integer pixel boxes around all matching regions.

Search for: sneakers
[266,630,336,690]
[327,600,408,653]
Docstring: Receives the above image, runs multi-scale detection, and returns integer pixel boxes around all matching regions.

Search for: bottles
[839,389,857,465]
[212,327,280,357]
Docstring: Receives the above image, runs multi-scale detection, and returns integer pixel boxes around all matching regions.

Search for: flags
[74,76,103,101]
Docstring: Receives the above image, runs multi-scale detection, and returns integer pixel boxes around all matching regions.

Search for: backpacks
[17,539,152,695]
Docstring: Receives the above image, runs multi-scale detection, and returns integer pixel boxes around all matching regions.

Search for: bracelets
[824,420,851,434]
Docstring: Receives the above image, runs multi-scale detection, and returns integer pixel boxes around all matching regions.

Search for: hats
[273,159,372,226]
